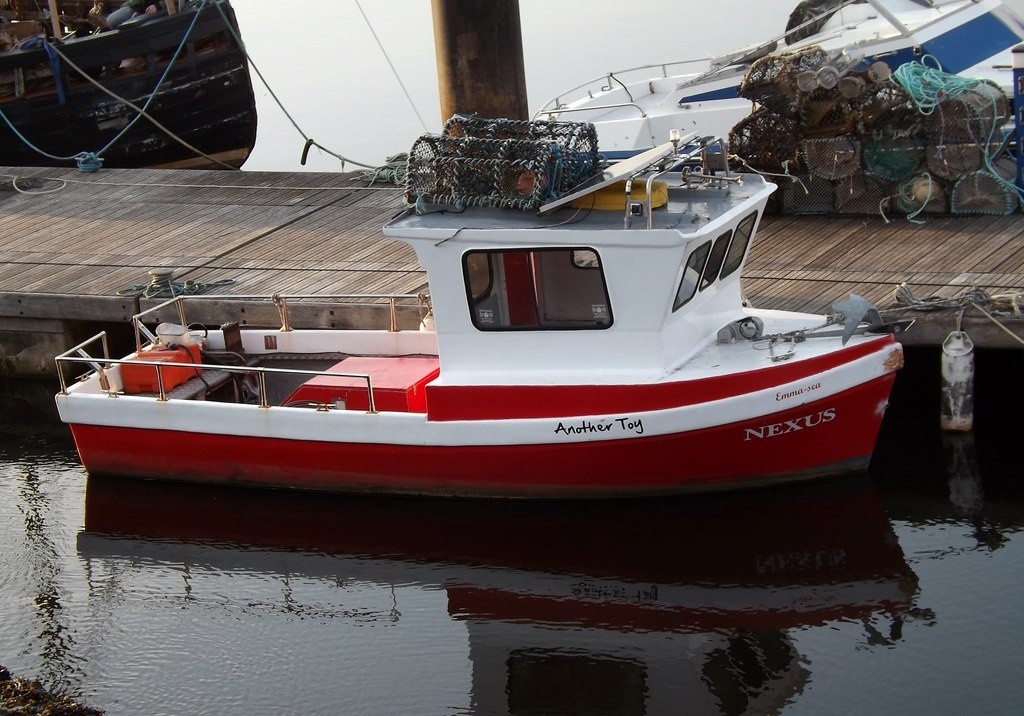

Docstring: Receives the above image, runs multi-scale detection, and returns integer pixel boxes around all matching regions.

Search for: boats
[529,1,1024,174]
[1,0,257,170]
[55,129,915,502]
